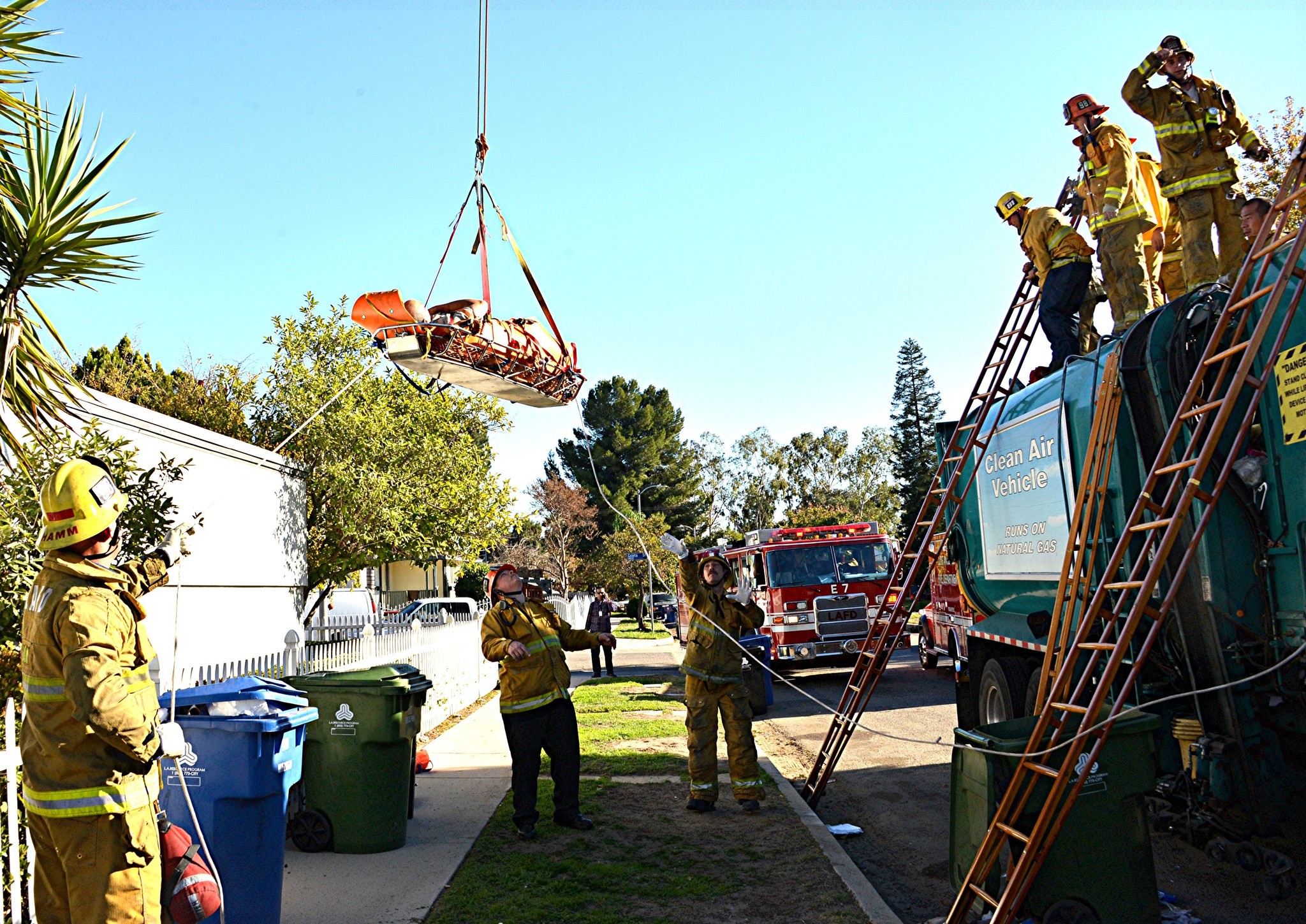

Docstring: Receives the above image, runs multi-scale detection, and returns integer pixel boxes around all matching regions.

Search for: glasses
[597,591,604,595]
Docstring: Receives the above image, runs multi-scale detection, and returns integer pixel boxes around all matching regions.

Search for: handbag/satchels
[156,818,220,924]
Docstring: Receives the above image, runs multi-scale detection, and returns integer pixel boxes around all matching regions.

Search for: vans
[304,588,379,653]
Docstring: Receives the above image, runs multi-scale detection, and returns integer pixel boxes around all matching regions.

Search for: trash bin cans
[156,674,319,924]
[735,635,774,715]
[944,702,1159,924]
[281,663,434,854]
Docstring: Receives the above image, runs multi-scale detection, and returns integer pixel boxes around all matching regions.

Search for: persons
[403,299,576,393]
[585,585,617,678]
[20,453,201,924]
[659,533,767,813]
[481,563,616,841]
[995,35,1273,380]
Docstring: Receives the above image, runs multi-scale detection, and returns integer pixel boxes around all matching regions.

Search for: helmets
[996,192,1034,223]
[34,458,130,551]
[483,564,517,607]
[1064,94,1110,126]
[698,548,731,581]
[1155,36,1195,76]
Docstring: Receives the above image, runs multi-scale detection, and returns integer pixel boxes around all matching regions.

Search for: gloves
[1066,196,1084,216]
[156,722,186,757]
[660,533,688,559]
[726,576,754,606]
[155,518,192,568]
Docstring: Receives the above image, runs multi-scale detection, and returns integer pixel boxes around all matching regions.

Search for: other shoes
[1111,329,1124,336]
[553,814,593,830]
[591,674,601,679]
[686,799,715,810]
[605,672,617,678]
[739,800,760,813]
[1040,357,1076,376]
[518,828,541,840]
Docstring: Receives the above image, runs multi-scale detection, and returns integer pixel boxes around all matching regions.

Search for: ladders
[939,129,1305,924]
[1032,351,1125,717]
[801,177,1085,810]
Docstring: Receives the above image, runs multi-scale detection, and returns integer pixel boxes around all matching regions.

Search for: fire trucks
[674,521,911,684]
[918,239,1306,907]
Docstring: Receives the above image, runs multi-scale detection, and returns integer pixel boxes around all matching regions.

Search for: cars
[647,592,677,619]
[343,597,479,641]
[618,594,630,606]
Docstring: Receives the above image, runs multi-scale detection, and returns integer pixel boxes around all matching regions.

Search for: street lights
[638,484,668,514]
